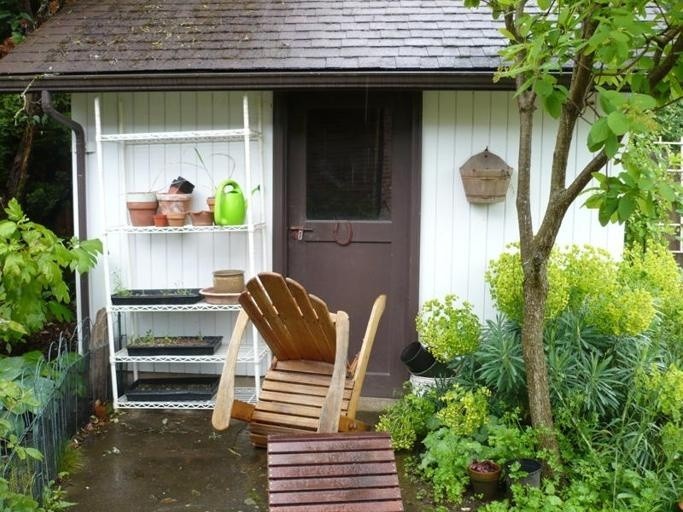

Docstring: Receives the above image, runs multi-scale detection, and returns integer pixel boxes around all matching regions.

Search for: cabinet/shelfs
[94,93,267,411]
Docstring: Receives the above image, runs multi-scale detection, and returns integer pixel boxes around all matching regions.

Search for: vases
[467,456,543,502]
[399,338,452,379]
[199,268,248,305]
[125,190,216,226]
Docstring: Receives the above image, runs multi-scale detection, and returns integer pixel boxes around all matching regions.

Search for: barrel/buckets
[459,152,513,205]
[418,361,456,380]
[408,374,457,394]
[399,342,434,371]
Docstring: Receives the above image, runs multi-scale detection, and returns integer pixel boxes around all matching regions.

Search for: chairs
[212,272,385,447]
